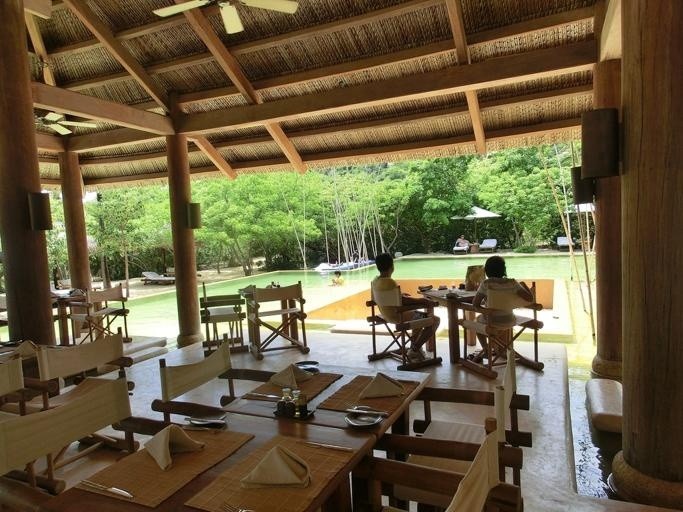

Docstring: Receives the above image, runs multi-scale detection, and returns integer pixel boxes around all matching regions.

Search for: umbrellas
[451,204,502,250]
[564,202,595,254]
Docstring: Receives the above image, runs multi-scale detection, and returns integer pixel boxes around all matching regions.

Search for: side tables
[575,239,593,252]
[468,242,480,253]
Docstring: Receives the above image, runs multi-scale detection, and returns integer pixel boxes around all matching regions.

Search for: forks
[220,501,255,512]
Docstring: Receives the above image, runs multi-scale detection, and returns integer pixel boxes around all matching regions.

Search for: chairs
[1,326,238,512]
[365,280,443,372]
[140,271,176,285]
[458,281,544,379]
[362,348,533,512]
[478,238,497,253]
[452,242,470,254]
[247,280,310,361]
[65,282,132,345]
[199,281,249,358]
[556,236,575,252]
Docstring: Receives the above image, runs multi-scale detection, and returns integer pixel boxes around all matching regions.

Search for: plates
[0,340,24,348]
[344,406,381,428]
[188,413,227,427]
[296,361,320,374]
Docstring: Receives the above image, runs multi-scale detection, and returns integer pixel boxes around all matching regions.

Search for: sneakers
[406,348,431,360]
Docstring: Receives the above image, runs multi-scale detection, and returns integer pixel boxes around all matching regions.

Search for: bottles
[449,285,459,295]
[281,388,301,411]
[458,284,465,295]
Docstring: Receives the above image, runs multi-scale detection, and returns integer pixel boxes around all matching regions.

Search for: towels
[143,363,405,489]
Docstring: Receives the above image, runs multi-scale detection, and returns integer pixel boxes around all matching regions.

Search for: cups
[298,394,308,414]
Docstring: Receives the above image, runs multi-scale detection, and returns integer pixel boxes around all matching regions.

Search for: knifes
[345,408,389,416]
[79,480,135,500]
[295,440,353,453]
[246,391,280,400]
[183,418,224,425]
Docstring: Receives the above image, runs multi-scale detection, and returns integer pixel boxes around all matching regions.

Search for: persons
[455,234,469,250]
[472,255,534,360]
[370,254,441,365]
[331,268,345,287]
